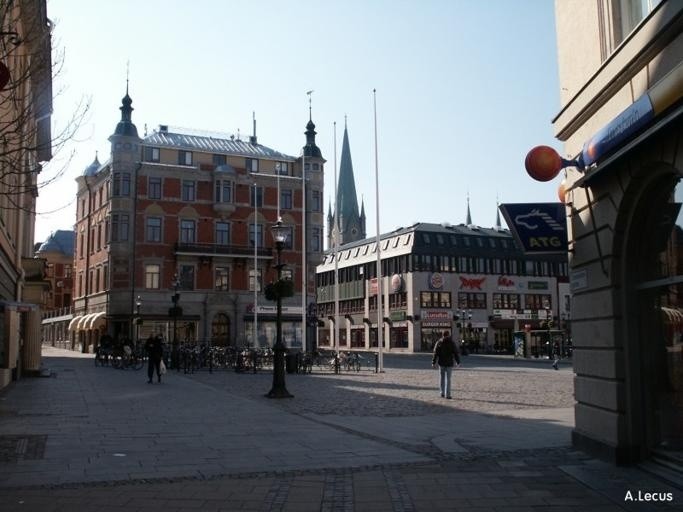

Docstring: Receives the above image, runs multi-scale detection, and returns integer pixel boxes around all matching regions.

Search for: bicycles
[93,347,364,372]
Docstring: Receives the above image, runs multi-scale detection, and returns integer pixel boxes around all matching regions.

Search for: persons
[432,331,461,400]
[144,330,165,383]
[552,338,560,370]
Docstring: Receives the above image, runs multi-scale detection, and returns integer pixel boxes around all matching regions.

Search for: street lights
[454,308,472,356]
[167,273,184,345]
[132,296,144,347]
[262,217,292,399]
